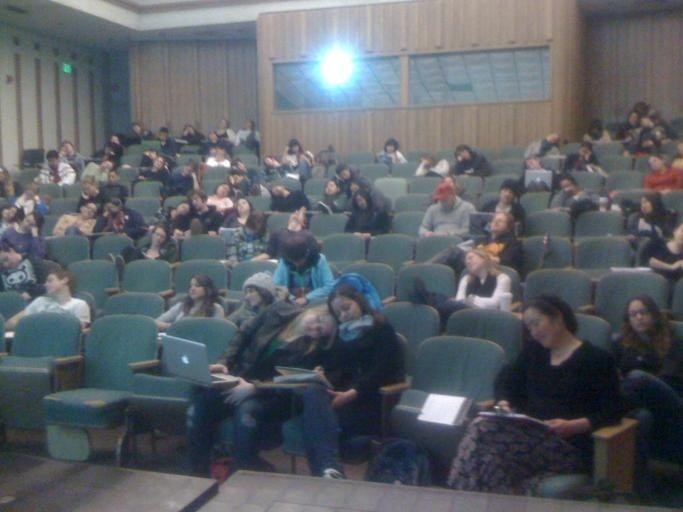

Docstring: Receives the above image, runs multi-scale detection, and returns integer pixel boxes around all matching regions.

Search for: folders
[476,405,549,429]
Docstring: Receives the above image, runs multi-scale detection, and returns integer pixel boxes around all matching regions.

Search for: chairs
[179,144,201,153]
[43,313,158,461]
[661,142,677,158]
[329,263,338,279]
[44,290,96,335]
[163,196,188,209]
[116,317,239,468]
[42,259,62,273]
[595,271,670,325]
[416,235,463,263]
[408,149,431,162]
[233,153,259,165]
[136,225,157,248]
[125,196,161,226]
[569,171,606,187]
[267,212,290,230]
[246,196,272,211]
[117,167,140,184]
[383,301,440,352]
[93,234,134,280]
[670,277,682,318]
[43,214,61,236]
[524,268,595,314]
[574,311,612,352]
[367,233,415,288]
[521,236,573,293]
[610,189,661,212]
[127,144,152,154]
[381,335,507,458]
[1,312,87,432]
[479,192,501,212]
[490,159,526,174]
[304,178,328,210]
[438,149,456,159]
[168,259,228,304]
[309,214,350,247]
[104,292,166,325]
[68,260,120,317]
[593,143,624,158]
[168,296,228,320]
[606,170,644,193]
[396,194,435,212]
[187,402,302,474]
[40,183,62,198]
[323,232,367,270]
[483,174,520,192]
[202,179,227,193]
[526,419,641,501]
[659,189,682,213]
[390,211,426,253]
[501,144,527,158]
[0,291,25,319]
[374,177,408,209]
[453,175,483,192]
[47,235,89,268]
[446,307,523,359]
[50,197,78,214]
[350,150,375,165]
[280,333,410,465]
[265,177,302,191]
[574,210,625,242]
[342,263,397,305]
[223,207,264,226]
[361,162,388,180]
[182,234,232,266]
[409,177,443,194]
[64,182,82,197]
[101,180,131,197]
[14,168,40,185]
[123,259,174,299]
[121,154,143,167]
[202,166,229,195]
[134,182,163,201]
[628,207,678,260]
[525,209,571,238]
[397,264,456,302]
[635,236,650,266]
[550,187,604,207]
[560,142,580,154]
[232,144,258,155]
[599,156,634,173]
[392,162,419,177]
[635,158,673,171]
[543,158,563,175]
[137,235,180,288]
[20,149,43,165]
[520,190,555,213]
[176,153,202,165]
[575,236,632,281]
[459,265,522,313]
[461,191,483,211]
[328,164,338,177]
[0,196,9,206]
[385,196,394,216]
[226,261,278,310]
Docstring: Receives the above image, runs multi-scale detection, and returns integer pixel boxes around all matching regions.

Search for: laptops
[162,333,239,389]
[524,169,552,191]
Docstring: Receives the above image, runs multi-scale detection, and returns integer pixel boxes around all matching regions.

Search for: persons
[99,171,129,204]
[671,140,683,169]
[180,125,204,146]
[270,183,310,228]
[0,214,48,260]
[583,121,611,143]
[93,197,148,239]
[631,103,664,127]
[634,191,679,241]
[204,145,232,168]
[228,172,261,196]
[521,157,560,194]
[283,138,315,167]
[374,139,409,176]
[524,133,564,158]
[205,131,232,156]
[455,144,491,176]
[207,181,234,214]
[305,282,407,480]
[315,180,348,214]
[0,240,48,299]
[379,250,512,331]
[642,222,683,279]
[141,151,159,169]
[641,151,683,190]
[78,159,115,186]
[474,178,527,237]
[262,156,292,182]
[35,151,78,186]
[57,141,86,176]
[163,160,200,196]
[2,204,21,236]
[55,202,103,238]
[174,191,223,240]
[274,233,335,307]
[423,175,474,217]
[419,183,477,239]
[106,223,179,282]
[91,143,123,165]
[155,273,225,344]
[651,127,676,151]
[231,159,259,186]
[550,173,608,211]
[103,133,125,153]
[345,190,391,248]
[151,128,177,155]
[15,184,50,215]
[235,121,260,151]
[4,267,92,336]
[224,271,292,330]
[335,162,371,189]
[74,176,109,214]
[139,156,171,184]
[634,133,660,158]
[182,300,339,480]
[121,123,143,146]
[415,153,450,179]
[565,141,606,172]
[421,211,520,273]
[618,110,651,156]
[214,117,235,142]
[0,166,23,197]
[613,294,683,496]
[442,293,623,497]
[146,201,195,239]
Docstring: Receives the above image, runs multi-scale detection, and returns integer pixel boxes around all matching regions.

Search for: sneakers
[320,468,345,480]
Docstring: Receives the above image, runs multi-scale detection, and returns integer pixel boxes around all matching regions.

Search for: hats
[242,270,276,298]
[434,183,454,199]
